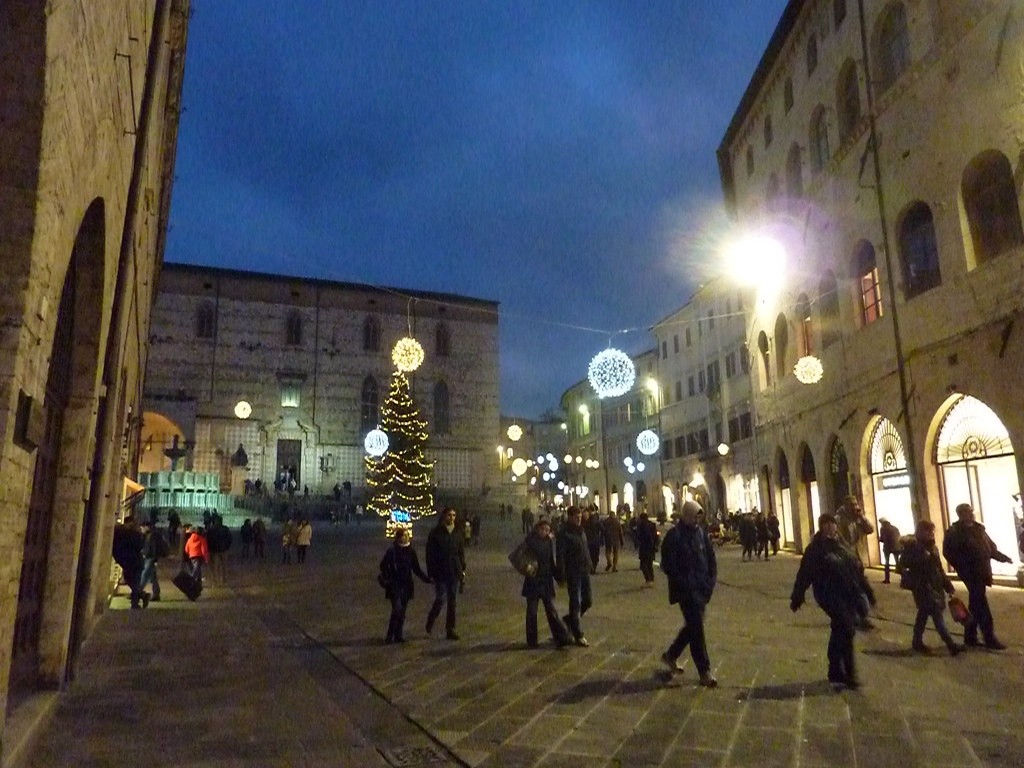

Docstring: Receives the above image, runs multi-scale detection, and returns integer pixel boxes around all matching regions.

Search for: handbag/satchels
[508,541,539,578]
[377,569,397,589]
[947,595,973,626]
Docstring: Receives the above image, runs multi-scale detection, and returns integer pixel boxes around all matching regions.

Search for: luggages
[168,569,205,602]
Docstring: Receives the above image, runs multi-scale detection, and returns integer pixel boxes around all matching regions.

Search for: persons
[557,506,594,648]
[790,495,876,689]
[280,517,313,565]
[379,529,433,646]
[356,502,364,526]
[902,504,1012,655]
[661,500,717,687]
[520,520,577,645]
[243,473,352,501]
[240,516,267,557]
[704,507,780,562]
[112,506,233,611]
[499,501,659,588]
[879,517,902,583]
[426,505,467,640]
[459,508,482,545]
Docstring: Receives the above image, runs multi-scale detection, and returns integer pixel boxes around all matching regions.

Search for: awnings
[122,478,146,511]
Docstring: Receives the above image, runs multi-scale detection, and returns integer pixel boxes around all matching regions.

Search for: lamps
[392,298,425,372]
[793,316,823,385]
[637,413,659,456]
[587,336,636,400]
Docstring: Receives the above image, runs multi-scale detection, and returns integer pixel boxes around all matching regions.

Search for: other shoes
[948,644,967,657]
[426,615,435,633]
[911,641,931,653]
[983,640,1006,652]
[963,639,985,648]
[445,627,459,639]
[661,651,684,674]
[575,637,590,647]
[142,592,151,609]
[700,673,717,686]
[149,596,161,601]
[384,634,393,645]
[562,615,575,632]
[131,604,142,611]
[394,636,406,644]
